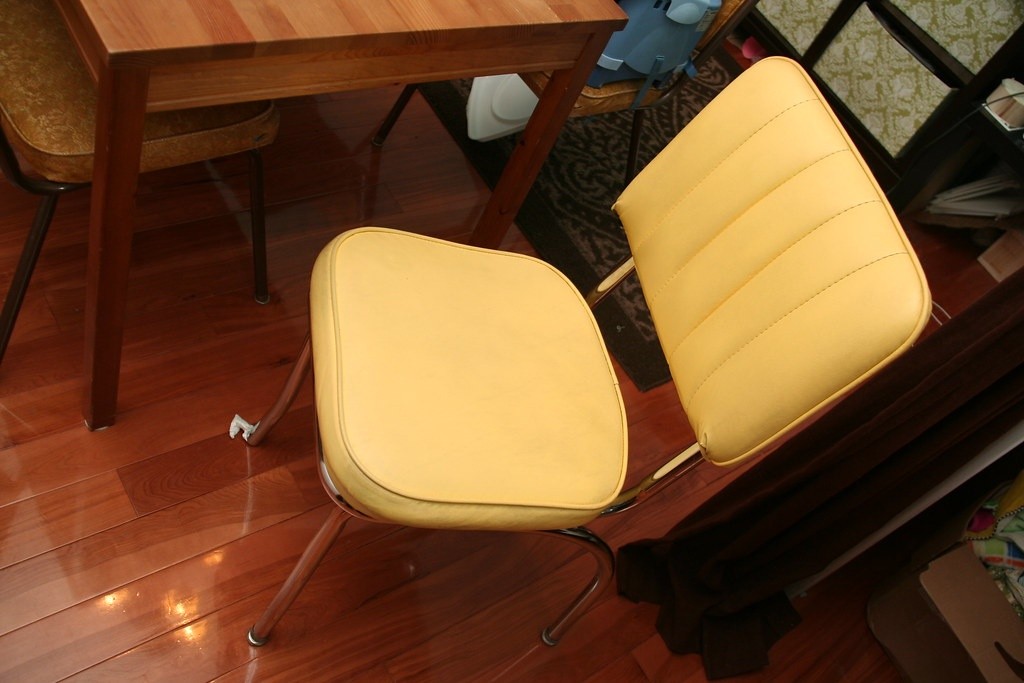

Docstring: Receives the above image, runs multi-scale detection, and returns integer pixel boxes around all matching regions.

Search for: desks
[55,0,630,432]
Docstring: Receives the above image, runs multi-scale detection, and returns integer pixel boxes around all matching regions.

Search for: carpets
[417,41,745,391]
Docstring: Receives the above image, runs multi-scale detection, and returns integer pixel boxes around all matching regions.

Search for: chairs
[372,0,759,230]
[0,1,280,364]
[249,55,934,647]
